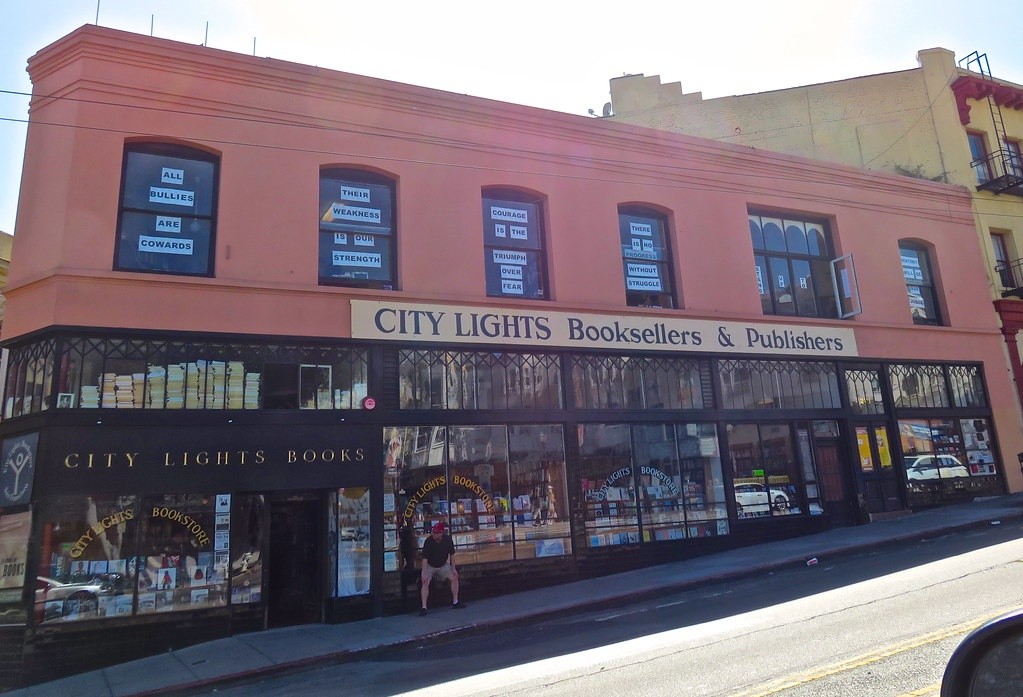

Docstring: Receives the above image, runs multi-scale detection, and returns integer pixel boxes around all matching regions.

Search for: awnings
[733,475,790,484]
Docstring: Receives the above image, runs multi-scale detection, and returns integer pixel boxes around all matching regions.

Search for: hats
[432,523,444,531]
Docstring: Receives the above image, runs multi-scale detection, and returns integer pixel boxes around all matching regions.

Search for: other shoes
[420,608,427,616]
[453,602,465,608]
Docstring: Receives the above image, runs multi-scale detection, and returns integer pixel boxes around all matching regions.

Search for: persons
[546,486,558,526]
[419,523,466,617]
[530,487,544,526]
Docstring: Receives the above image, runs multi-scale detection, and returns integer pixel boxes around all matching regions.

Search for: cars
[715,483,791,515]
[903,454,970,489]
[35,577,110,622]
[342,528,357,540]
